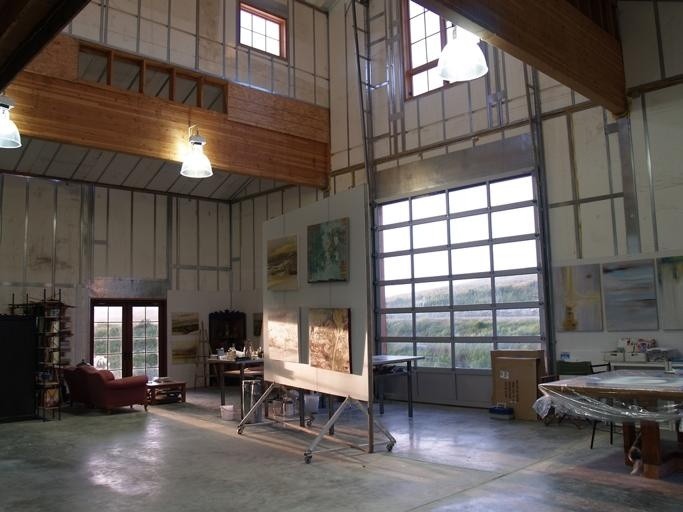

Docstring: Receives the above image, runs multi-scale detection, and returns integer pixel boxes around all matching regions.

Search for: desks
[297,355,426,434]
[610,360,683,371]
[207,359,286,419]
[538,373,683,478]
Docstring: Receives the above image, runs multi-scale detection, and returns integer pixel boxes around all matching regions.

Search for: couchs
[79,365,149,414]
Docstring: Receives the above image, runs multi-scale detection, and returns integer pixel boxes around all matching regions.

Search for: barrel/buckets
[304,394,319,413]
[220,405,233,421]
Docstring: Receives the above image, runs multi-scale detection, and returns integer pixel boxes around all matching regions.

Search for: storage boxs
[491,349,545,416]
[494,357,538,421]
[489,402,514,421]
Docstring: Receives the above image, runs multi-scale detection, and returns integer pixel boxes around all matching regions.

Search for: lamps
[436,25,488,86]
[180,124,214,179]
[0,95,24,149]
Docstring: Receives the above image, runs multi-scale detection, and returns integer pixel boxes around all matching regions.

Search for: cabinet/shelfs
[30,285,74,421]
[0,315,38,419]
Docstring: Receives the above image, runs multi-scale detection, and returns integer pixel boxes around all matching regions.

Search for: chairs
[557,360,614,449]
